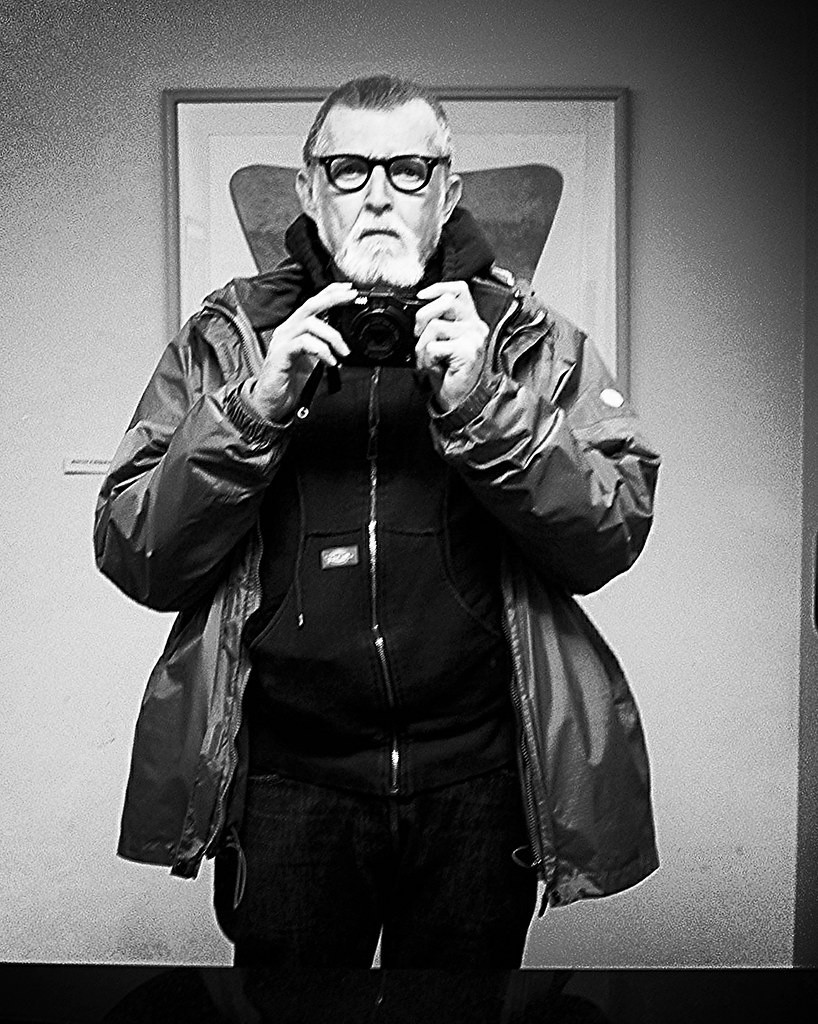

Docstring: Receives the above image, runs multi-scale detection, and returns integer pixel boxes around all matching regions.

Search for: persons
[93,76,663,970]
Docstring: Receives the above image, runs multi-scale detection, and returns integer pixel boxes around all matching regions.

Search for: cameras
[329,286,438,370]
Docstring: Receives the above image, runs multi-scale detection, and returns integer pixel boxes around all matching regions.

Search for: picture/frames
[164,86,628,397]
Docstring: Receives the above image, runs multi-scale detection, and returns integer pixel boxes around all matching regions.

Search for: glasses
[307,154,451,194]
